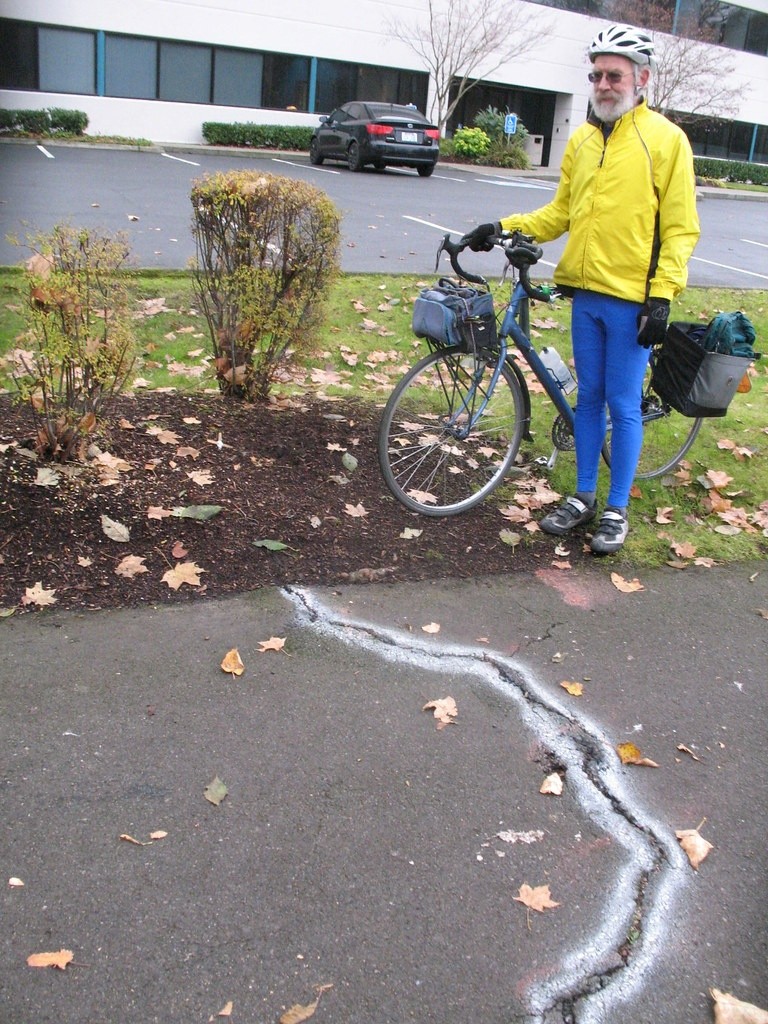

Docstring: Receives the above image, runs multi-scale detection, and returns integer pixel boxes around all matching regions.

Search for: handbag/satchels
[411,276,497,353]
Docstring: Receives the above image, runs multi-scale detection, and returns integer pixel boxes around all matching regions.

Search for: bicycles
[374,228,704,517]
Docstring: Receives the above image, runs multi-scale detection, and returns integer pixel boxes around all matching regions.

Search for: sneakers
[541,492,629,553]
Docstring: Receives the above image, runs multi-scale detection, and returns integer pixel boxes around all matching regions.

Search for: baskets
[650,321,762,418]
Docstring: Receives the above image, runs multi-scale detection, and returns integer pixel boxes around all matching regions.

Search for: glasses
[588,71,634,84]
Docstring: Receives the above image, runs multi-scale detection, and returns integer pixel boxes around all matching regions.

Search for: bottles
[540,346,576,394]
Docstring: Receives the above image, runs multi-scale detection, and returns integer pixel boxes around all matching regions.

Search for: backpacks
[700,309,756,358]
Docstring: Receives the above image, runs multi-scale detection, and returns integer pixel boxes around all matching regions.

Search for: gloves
[459,221,502,252]
[635,297,672,349]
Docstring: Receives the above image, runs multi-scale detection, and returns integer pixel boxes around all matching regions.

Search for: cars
[308,101,439,178]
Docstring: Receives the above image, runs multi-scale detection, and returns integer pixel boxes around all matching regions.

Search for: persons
[457,24,702,555]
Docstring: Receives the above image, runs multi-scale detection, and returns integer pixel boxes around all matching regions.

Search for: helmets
[588,23,655,70]
